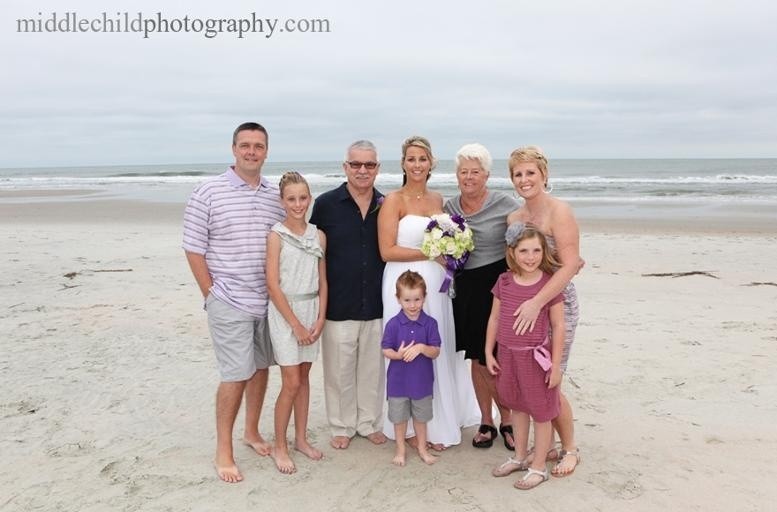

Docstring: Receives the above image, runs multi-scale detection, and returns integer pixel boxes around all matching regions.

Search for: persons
[378,135,449,454]
[380,270,442,468]
[308,140,388,449]
[181,122,287,483]
[507,146,579,477]
[444,144,586,451]
[486,222,565,490]
[267,171,328,475]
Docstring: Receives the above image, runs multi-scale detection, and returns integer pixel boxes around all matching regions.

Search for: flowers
[415,212,477,271]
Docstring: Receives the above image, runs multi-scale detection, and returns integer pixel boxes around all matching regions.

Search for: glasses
[346,160,377,169]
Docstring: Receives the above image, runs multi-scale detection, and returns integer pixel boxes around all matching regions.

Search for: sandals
[493,446,580,490]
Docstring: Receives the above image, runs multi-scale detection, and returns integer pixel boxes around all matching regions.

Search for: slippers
[472,424,497,448]
[499,423,515,450]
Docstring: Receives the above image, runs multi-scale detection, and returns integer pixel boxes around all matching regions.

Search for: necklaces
[401,186,430,200]
[460,187,487,211]
